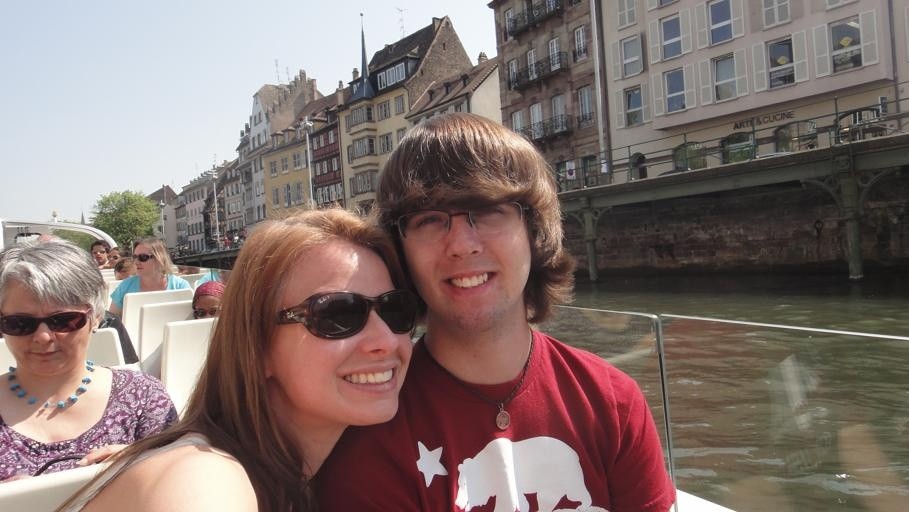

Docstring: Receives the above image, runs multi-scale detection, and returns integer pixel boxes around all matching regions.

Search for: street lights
[128,238,134,258]
[298,114,316,211]
[159,199,164,239]
[209,167,221,251]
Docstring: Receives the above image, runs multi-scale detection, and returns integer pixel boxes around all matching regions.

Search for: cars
[807,118,886,151]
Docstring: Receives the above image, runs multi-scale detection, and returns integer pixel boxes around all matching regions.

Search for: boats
[0,218,909,511]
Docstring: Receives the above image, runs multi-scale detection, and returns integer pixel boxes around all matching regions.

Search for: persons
[1,237,181,483]
[34,235,227,319]
[48,199,423,512]
[312,113,676,512]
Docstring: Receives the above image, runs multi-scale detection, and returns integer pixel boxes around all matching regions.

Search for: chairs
[157,317,220,423]
[138,299,194,364]
[120,286,195,357]
[0,327,125,376]
[1,460,119,512]
[99,268,122,312]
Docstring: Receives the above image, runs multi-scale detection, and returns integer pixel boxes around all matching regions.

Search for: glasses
[93,250,106,254]
[250,287,417,335]
[109,255,119,259]
[194,309,221,318]
[388,200,529,242]
[0,307,93,337]
[130,253,154,262]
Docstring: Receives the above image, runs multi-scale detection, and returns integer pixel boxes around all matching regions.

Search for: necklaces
[420,332,535,430]
[7,357,97,407]
